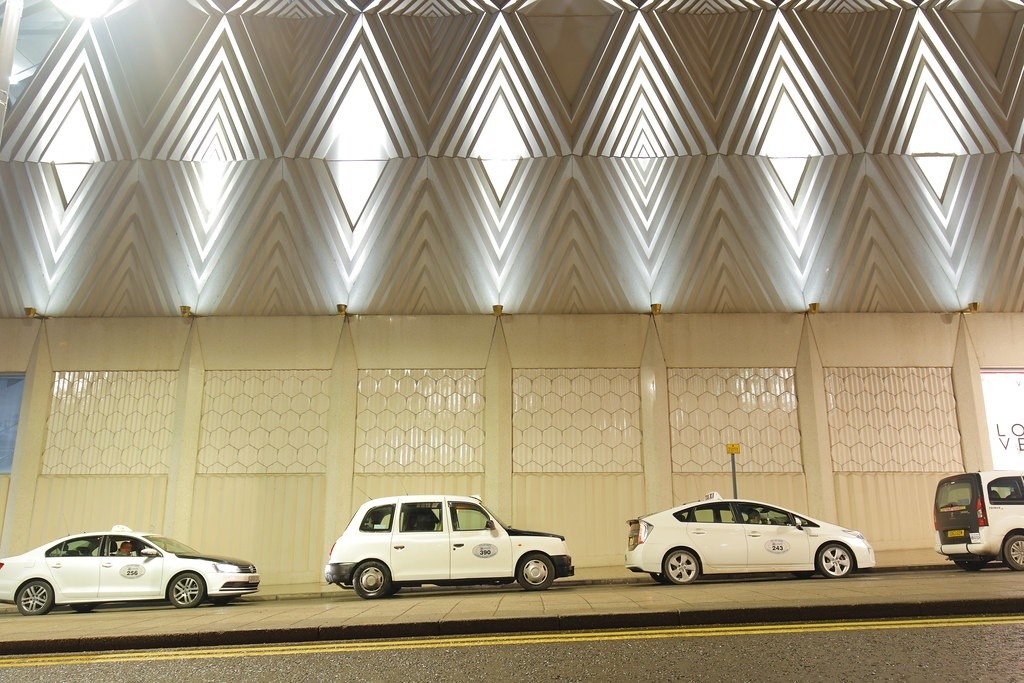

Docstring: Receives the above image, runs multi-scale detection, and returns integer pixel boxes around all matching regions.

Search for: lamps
[806,303,820,315]
[650,304,661,315]
[180,306,201,318]
[337,304,352,317]
[960,302,981,315]
[493,305,509,316]
[24,308,48,319]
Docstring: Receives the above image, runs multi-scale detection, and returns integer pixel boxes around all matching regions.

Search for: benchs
[59,544,92,556]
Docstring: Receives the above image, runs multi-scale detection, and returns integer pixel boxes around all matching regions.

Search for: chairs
[110,541,117,556]
[990,491,1001,500]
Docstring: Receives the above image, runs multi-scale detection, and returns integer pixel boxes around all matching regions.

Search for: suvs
[322,493,577,600]
[933,469,1024,574]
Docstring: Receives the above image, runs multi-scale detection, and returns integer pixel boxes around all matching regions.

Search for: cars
[625,491,878,587]
[0,523,262,616]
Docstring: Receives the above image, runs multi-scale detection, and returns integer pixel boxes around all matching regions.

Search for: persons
[745,507,777,525]
[116,541,132,557]
